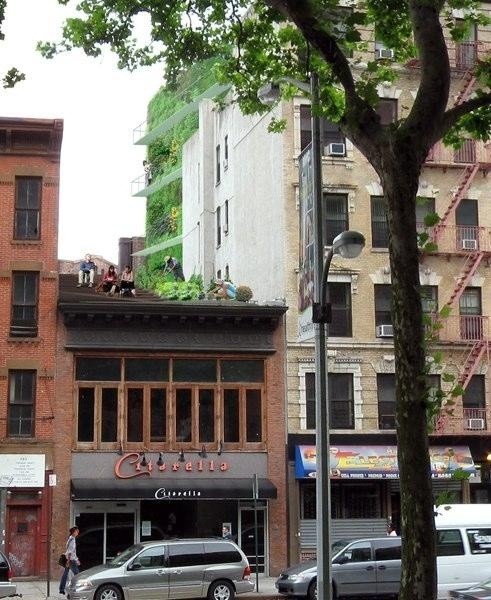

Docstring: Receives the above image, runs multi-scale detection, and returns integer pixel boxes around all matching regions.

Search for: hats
[164,256,170,263]
[213,279,223,285]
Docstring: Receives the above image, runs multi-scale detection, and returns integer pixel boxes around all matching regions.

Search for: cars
[0,551,17,597]
[449,580,491,599]
[227,521,264,559]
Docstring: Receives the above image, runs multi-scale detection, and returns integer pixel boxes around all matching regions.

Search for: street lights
[310,67,368,600]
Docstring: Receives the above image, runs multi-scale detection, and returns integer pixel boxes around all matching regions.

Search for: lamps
[116,439,227,468]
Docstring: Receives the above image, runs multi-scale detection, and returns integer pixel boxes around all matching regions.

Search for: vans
[66,536,254,599]
[275,535,403,600]
[431,503,491,600]
[72,522,164,569]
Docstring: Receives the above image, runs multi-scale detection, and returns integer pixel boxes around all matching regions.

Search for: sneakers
[76,284,82,287]
[88,282,93,287]
[59,590,65,596]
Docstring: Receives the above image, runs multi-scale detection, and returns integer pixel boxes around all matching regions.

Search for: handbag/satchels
[57,554,73,569]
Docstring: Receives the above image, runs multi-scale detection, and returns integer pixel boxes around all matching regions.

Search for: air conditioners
[377,47,395,62]
[467,418,485,431]
[326,141,346,156]
[377,324,395,338]
[462,237,478,250]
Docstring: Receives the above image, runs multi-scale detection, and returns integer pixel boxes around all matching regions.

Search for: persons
[386,524,397,537]
[120,265,136,298]
[77,253,98,288]
[162,254,186,282]
[157,513,179,540]
[59,526,82,592]
[212,279,236,300]
[221,524,231,543]
[101,264,119,297]
